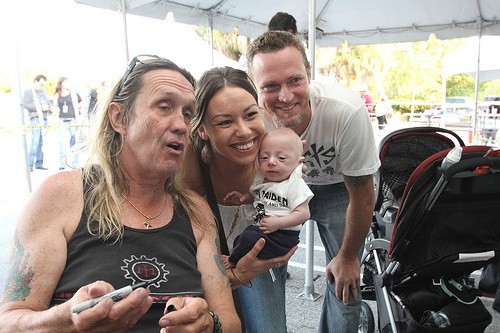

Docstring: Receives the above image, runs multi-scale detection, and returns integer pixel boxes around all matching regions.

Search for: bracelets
[208,310,222,333]
[229,267,252,289]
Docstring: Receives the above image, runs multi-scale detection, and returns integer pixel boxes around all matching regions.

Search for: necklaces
[115,185,167,229]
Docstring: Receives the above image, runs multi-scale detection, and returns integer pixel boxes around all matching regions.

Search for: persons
[245,31,381,333]
[22,74,99,171]
[180,66,307,333]
[221,126,314,269]
[0,53,244,333]
[268,12,299,37]
[358,84,391,129]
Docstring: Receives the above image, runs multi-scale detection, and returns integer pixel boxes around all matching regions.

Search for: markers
[72,281,148,313]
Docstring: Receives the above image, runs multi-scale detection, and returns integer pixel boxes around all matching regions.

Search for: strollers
[359,145,499,333]
[359,126,466,287]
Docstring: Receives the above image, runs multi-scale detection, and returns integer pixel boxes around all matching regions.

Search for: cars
[439,96,500,121]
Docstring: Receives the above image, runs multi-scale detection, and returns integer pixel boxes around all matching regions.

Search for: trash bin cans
[480,128,499,145]
[430,116,441,127]
[376,115,387,129]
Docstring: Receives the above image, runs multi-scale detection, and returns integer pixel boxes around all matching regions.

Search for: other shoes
[36,167,48,170]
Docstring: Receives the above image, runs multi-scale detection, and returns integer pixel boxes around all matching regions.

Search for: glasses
[117,55,162,96]
